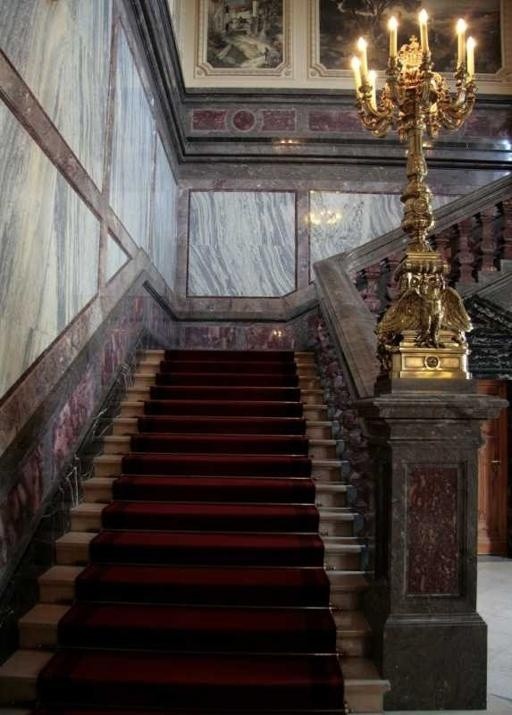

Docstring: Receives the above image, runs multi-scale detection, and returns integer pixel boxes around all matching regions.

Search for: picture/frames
[194,0,295,80]
[306,0,511,82]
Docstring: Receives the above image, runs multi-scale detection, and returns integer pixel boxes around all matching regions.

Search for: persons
[415,284,432,348]
[415,280,447,349]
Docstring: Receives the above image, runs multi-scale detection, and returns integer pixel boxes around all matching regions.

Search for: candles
[367,68,378,110]
[417,8,431,55]
[349,56,362,96]
[465,34,477,77]
[454,17,468,69]
[387,15,399,56]
[355,37,369,85]
